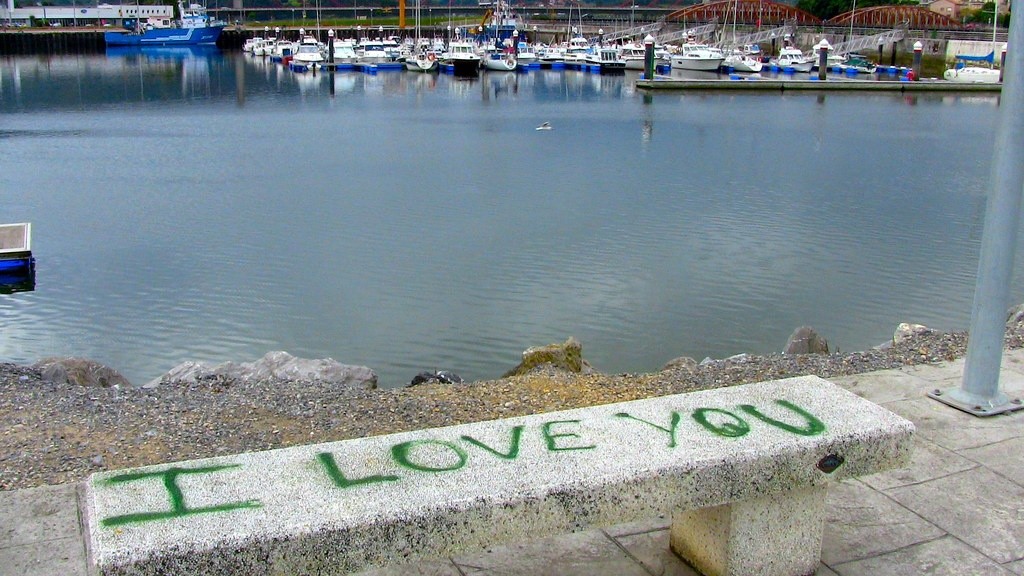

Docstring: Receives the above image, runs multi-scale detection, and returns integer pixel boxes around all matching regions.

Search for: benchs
[70,373,915,576]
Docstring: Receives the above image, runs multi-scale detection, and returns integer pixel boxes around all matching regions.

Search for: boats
[0,223,36,280]
[105,3,228,47]
[243,26,905,74]
[943,65,1001,84]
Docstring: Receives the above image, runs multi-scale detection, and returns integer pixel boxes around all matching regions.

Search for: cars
[533,13,594,20]
[2,21,112,28]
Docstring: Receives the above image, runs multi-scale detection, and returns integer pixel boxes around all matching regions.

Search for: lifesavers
[427,52,435,62]
